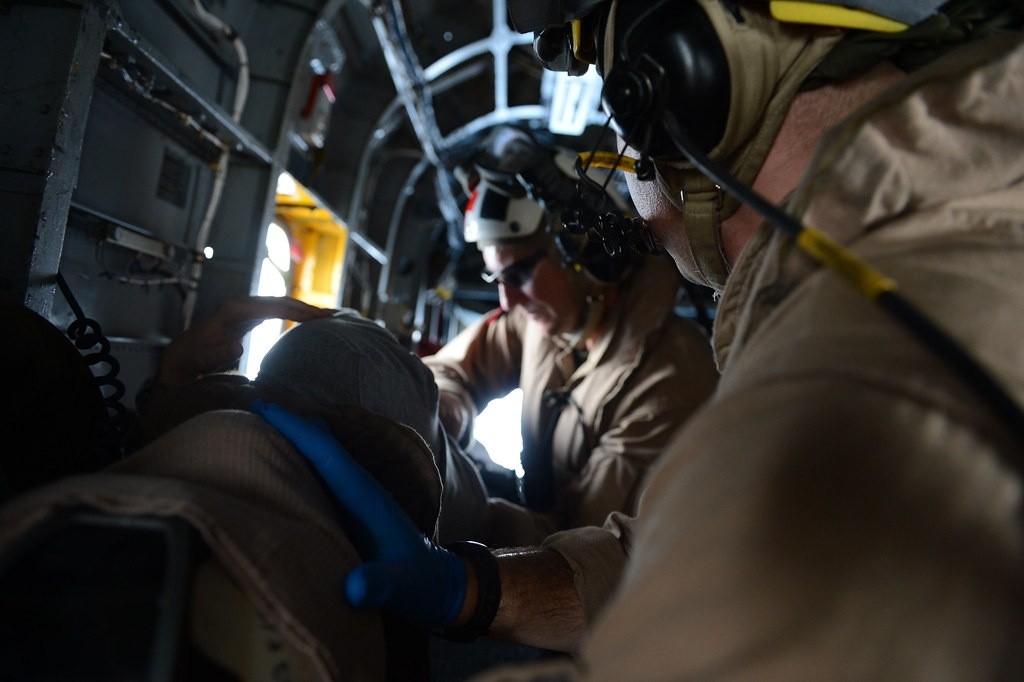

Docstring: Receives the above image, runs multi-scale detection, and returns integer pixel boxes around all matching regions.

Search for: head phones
[537,0,731,183]
[479,126,648,285]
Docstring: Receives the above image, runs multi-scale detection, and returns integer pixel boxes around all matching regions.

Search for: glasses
[480,239,554,290]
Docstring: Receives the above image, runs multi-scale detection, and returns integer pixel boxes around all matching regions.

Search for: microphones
[562,113,658,262]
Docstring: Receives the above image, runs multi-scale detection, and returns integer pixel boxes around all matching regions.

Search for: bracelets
[441,540,501,644]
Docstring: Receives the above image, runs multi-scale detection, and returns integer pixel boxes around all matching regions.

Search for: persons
[78,0,1024,682]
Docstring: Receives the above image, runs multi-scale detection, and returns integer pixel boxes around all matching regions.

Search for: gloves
[245,396,466,624]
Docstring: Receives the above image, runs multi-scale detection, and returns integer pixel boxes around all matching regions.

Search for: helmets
[463,144,581,243]
